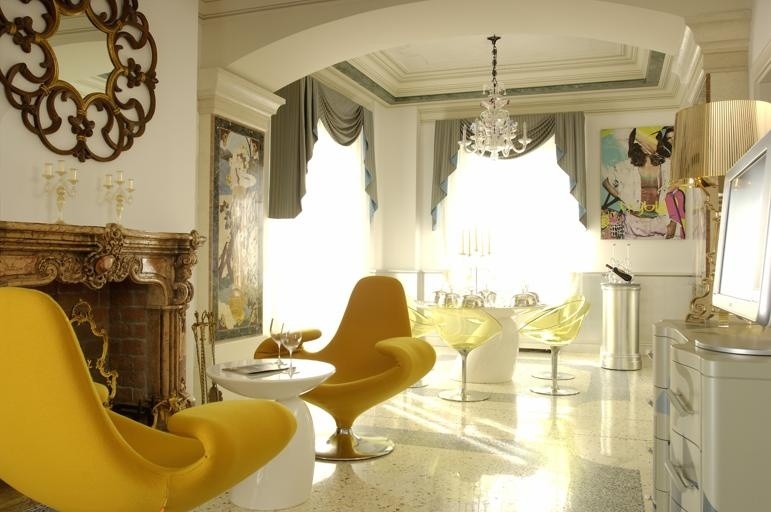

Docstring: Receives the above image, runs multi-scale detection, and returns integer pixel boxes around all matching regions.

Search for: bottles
[605,263,632,282]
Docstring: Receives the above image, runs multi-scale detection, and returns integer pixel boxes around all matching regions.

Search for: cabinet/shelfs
[654,319,770,512]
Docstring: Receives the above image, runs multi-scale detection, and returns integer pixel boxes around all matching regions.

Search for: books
[220,361,295,377]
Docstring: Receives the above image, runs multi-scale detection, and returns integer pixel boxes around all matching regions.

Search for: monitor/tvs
[694,131,771,356]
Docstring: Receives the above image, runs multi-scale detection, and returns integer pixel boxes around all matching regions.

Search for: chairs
[519,294,586,380]
[407,303,431,388]
[519,293,592,396]
[0,286,298,512]
[253,275,437,461]
[431,305,503,402]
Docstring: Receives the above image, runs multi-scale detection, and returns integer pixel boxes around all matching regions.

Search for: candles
[42,159,135,192]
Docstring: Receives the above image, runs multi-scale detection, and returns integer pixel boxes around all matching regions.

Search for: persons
[654,125,675,161]
[602,128,680,241]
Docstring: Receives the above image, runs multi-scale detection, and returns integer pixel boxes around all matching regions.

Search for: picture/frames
[209,114,267,343]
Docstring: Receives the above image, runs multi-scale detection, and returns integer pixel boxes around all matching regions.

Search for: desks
[205,357,337,510]
[416,303,548,384]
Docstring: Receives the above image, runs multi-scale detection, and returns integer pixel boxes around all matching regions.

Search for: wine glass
[280,321,303,376]
[270,318,288,366]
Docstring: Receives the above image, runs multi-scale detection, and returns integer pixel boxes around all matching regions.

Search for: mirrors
[0,0,158,163]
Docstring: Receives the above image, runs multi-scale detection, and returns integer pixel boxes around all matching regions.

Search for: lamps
[458,34,533,162]
[669,100,771,322]
